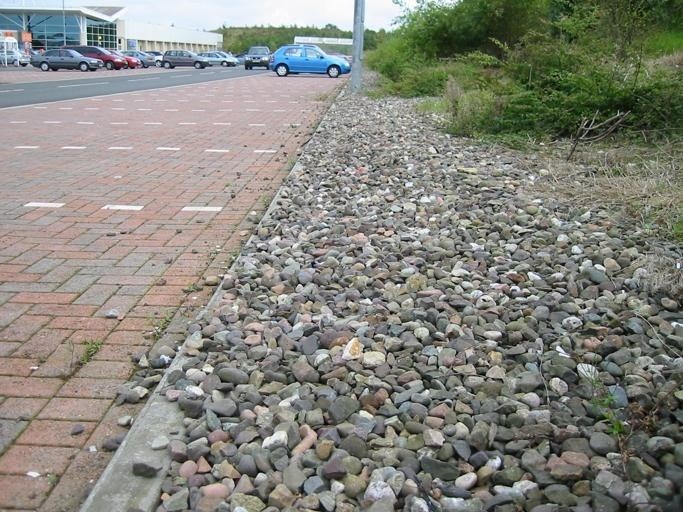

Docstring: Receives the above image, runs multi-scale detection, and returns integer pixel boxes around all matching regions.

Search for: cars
[197,51,240,67]
[161,49,210,69]
[0,49,31,67]
[107,48,164,70]
[30,49,104,73]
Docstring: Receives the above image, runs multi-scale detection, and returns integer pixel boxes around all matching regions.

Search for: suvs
[268,44,352,78]
[244,46,273,70]
[61,45,129,71]
